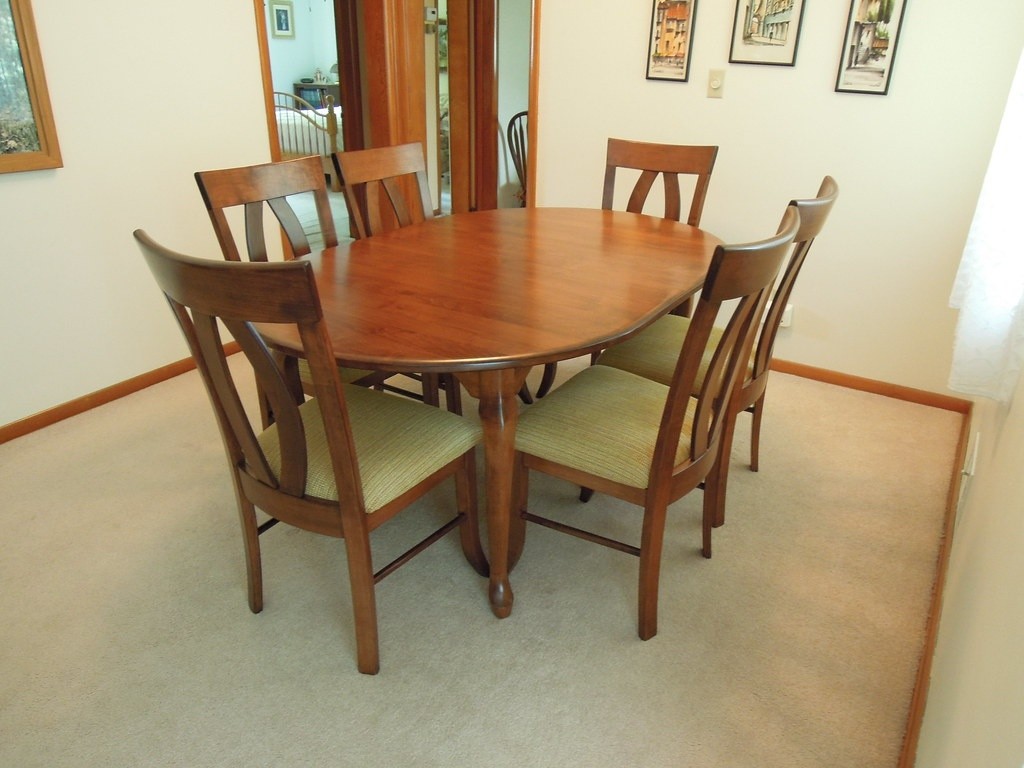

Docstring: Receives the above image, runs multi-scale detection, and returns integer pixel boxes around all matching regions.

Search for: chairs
[507,110,528,207]
[506,205,801,641]
[601,138,718,318]
[195,141,463,417]
[133,229,489,675]
[595,175,838,528]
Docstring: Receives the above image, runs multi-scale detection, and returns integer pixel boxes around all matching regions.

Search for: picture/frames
[268,0,296,40]
[439,18,448,72]
[728,0,807,67]
[645,0,698,83]
[0,1,64,174]
[835,0,908,95]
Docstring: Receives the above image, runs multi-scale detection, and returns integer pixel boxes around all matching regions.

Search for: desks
[253,208,725,619]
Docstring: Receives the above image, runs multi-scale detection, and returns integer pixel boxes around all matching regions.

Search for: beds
[274,91,345,192]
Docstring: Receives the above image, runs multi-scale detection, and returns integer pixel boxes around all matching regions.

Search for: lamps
[331,64,340,84]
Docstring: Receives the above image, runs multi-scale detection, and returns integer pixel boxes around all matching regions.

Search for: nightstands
[293,82,341,110]
